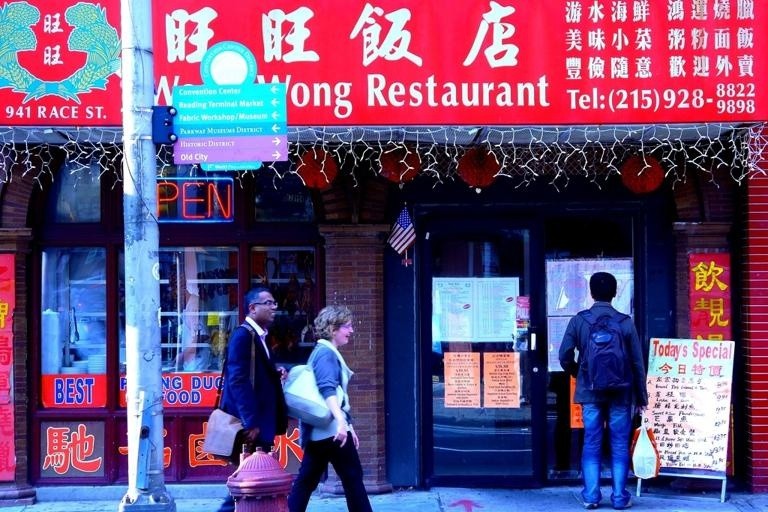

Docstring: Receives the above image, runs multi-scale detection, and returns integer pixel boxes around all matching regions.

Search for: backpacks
[578,308,634,394]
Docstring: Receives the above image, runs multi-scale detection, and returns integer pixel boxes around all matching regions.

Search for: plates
[61,355,108,374]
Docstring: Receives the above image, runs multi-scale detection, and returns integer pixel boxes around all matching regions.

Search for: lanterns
[375,145,420,180]
[619,153,664,193]
[296,147,335,190]
[457,146,500,187]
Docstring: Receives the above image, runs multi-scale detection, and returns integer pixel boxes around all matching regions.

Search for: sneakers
[615,498,632,510]
[583,499,599,509]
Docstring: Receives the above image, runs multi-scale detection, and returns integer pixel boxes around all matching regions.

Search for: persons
[286,304,373,512]
[558,271,649,509]
[212,289,288,512]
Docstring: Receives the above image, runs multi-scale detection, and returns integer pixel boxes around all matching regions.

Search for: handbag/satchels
[202,409,247,465]
[633,423,661,480]
[283,365,345,427]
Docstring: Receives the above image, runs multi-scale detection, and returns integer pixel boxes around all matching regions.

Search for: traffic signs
[171,83,287,166]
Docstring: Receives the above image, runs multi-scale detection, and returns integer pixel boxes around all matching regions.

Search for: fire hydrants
[226,444,298,512]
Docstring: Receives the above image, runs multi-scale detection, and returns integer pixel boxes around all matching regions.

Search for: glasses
[337,323,352,330]
[255,301,278,308]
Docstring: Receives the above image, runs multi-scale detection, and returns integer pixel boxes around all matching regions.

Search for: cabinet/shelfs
[61,277,322,374]
